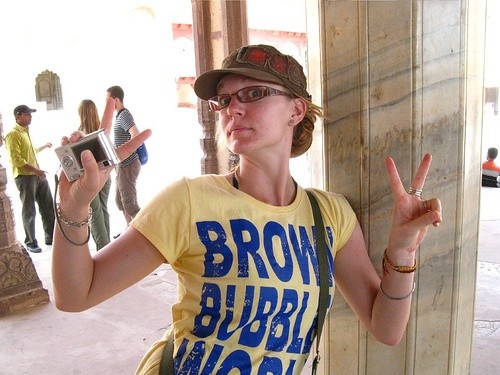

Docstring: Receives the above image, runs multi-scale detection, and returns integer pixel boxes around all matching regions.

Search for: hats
[193,44,312,102]
[14,105,36,116]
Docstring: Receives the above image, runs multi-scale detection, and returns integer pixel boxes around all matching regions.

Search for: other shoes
[113,234,120,239]
[45,242,52,245]
[27,245,42,253]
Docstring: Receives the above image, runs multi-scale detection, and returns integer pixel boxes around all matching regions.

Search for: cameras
[53,127,121,183]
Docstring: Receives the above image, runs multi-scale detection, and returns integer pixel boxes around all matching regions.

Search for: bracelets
[56,203,93,228]
[382,248,417,276]
[380,279,415,301]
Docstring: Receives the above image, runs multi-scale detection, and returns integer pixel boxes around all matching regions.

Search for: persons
[52,45,442,375]
[77,99,111,251]
[106,86,142,239]
[482,148,500,188]
[4,105,57,253]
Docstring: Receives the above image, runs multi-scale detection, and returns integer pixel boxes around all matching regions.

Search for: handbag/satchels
[137,143,149,165]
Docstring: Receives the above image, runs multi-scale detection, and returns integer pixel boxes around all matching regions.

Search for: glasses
[207,85,295,111]
